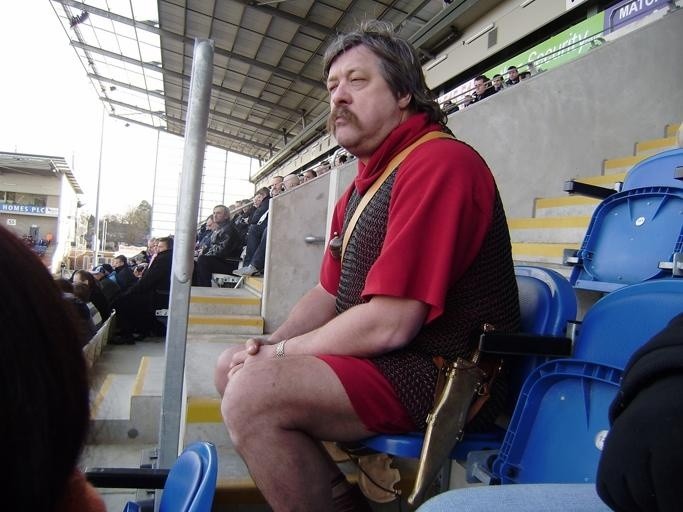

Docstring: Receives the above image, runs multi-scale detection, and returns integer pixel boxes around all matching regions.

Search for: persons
[1,225,108,511]
[21,234,50,256]
[188,153,349,286]
[214,18,524,511]
[441,66,530,117]
[55,235,175,350]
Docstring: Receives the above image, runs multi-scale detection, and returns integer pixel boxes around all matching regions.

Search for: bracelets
[277,340,286,357]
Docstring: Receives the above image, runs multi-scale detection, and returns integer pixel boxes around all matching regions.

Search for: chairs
[80,204,264,345]
[561,145,682,297]
[466,278,683,487]
[340,264,578,512]
[79,439,219,511]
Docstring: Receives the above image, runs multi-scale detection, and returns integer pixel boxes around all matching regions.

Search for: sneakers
[233,265,261,277]
[109,334,135,346]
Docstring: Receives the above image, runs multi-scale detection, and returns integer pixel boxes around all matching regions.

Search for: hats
[92,266,106,274]
